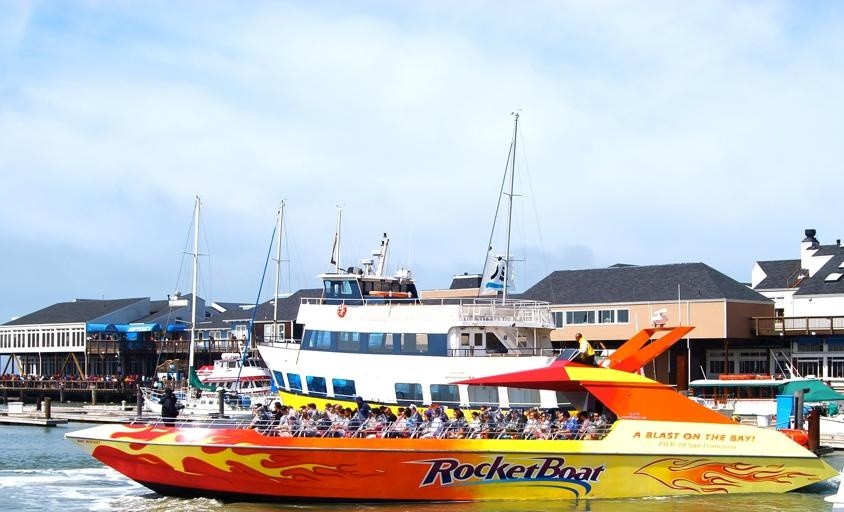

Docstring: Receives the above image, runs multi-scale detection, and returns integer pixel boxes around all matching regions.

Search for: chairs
[251,409,588,442]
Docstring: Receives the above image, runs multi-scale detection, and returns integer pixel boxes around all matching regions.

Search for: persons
[356,397,372,419]
[247,401,616,438]
[230,333,237,348]
[242,334,246,344]
[575,333,596,365]
[158,388,180,427]
[209,335,215,350]
[0,370,176,390]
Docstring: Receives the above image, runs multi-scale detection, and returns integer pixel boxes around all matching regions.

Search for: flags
[479,256,515,298]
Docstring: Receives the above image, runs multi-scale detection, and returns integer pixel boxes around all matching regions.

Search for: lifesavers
[337,304,348,318]
